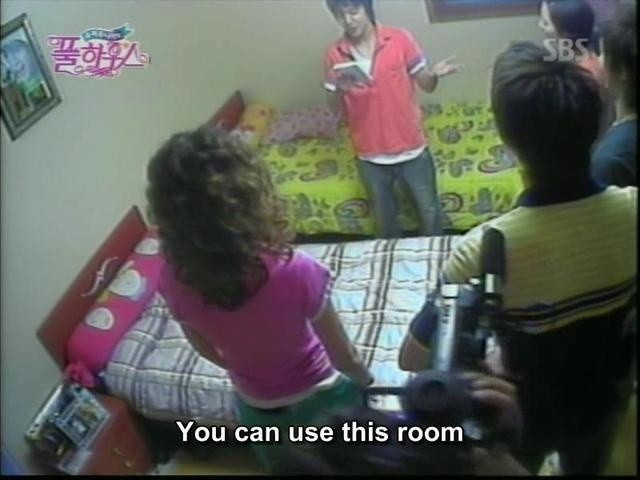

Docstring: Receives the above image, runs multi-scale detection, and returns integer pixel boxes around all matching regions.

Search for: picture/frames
[24,382,79,463]
[0,13,61,142]
[55,389,110,449]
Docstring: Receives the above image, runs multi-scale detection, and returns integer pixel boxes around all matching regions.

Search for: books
[332,62,369,85]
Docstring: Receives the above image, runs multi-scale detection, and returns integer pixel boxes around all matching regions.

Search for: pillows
[259,107,349,146]
[64,230,169,388]
[229,103,276,155]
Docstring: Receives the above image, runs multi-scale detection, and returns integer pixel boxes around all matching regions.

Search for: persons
[398,0,639,475]
[271,370,531,475]
[1,39,32,80]
[144,126,375,474]
[323,1,465,240]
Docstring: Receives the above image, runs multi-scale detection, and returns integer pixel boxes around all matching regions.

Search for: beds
[200,89,526,236]
[36,206,502,463]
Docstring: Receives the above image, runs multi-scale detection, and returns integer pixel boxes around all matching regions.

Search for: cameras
[400,225,510,436]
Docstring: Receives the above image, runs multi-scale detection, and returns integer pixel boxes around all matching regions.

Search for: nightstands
[39,389,157,475]
